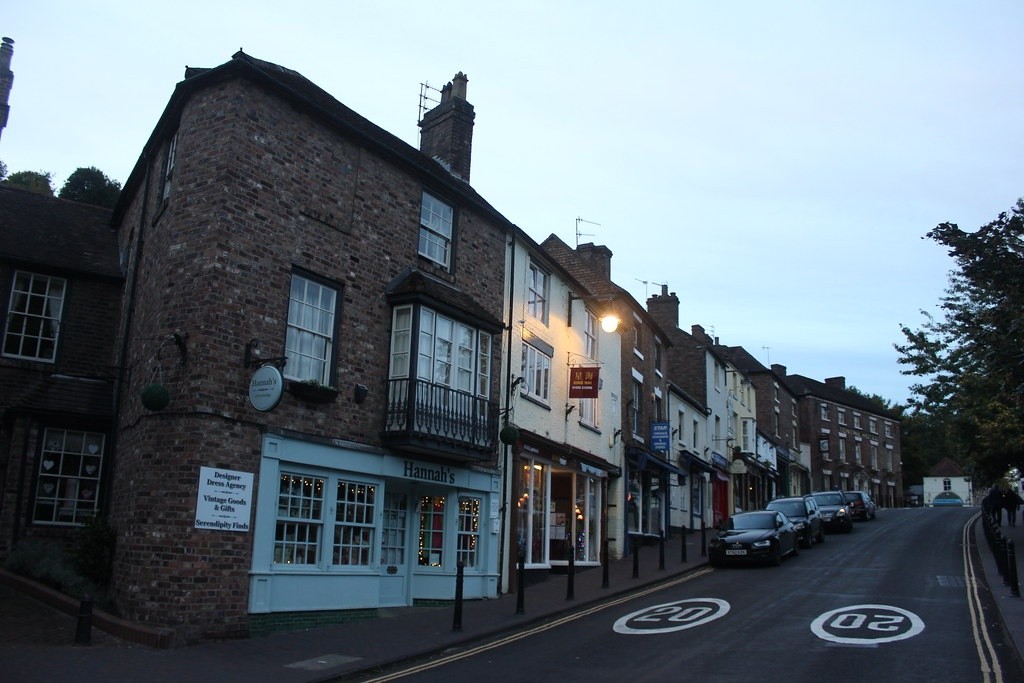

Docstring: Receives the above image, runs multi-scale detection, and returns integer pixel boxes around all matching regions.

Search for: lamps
[567,290,619,334]
[724,368,752,389]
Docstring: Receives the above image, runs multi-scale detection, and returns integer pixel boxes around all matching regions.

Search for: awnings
[626,446,685,476]
[679,449,717,475]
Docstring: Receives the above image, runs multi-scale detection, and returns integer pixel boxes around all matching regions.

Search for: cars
[708,510,800,566]
[843,491,877,522]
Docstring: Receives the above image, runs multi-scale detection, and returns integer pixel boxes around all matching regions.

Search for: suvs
[808,490,854,534]
[766,494,824,548]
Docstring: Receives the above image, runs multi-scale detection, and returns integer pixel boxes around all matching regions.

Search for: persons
[981,484,1019,527]
